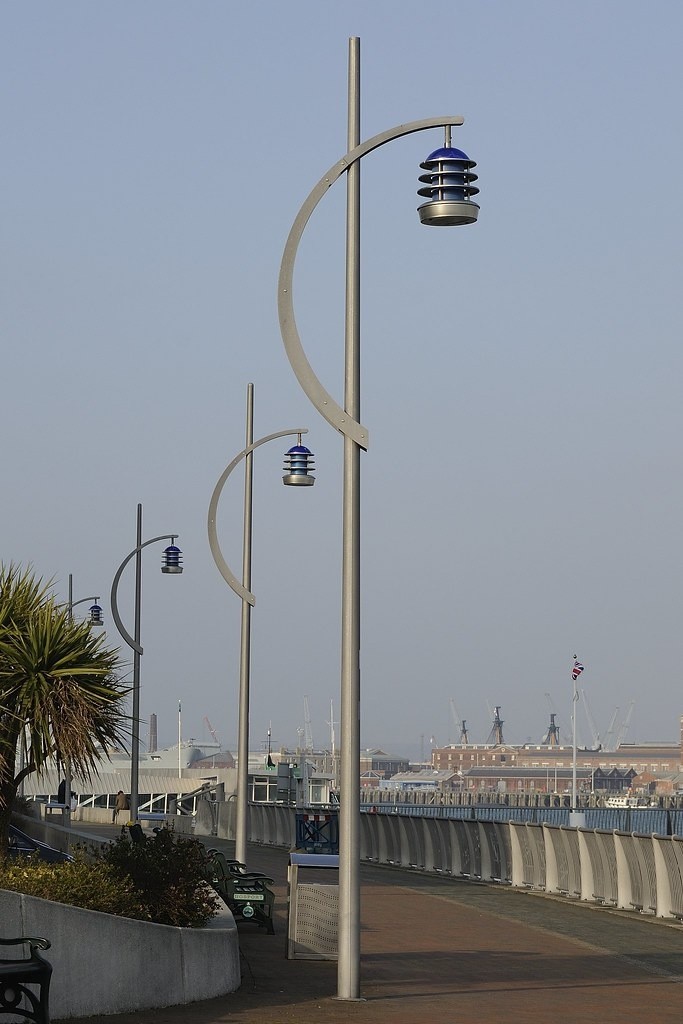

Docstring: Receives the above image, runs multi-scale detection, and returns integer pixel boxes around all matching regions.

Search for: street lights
[279,36,482,1004]
[59,573,108,825]
[113,502,182,839]
[207,385,317,895]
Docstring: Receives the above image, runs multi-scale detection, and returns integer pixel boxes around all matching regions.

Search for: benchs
[207,848,274,935]
[0,938,53,1024]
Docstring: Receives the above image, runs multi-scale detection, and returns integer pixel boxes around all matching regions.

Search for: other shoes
[111,821,114,824]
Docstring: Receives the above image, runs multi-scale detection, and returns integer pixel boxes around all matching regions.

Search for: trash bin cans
[135,813,168,837]
[284,852,340,962]
[45,803,71,828]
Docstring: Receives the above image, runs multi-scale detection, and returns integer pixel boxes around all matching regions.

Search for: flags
[494,708,497,716]
[430,737,434,744]
[572,661,584,680]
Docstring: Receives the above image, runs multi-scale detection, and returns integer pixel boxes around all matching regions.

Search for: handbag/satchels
[71,796,77,812]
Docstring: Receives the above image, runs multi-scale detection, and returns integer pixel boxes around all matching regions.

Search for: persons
[58,775,76,815]
[110,790,130,824]
